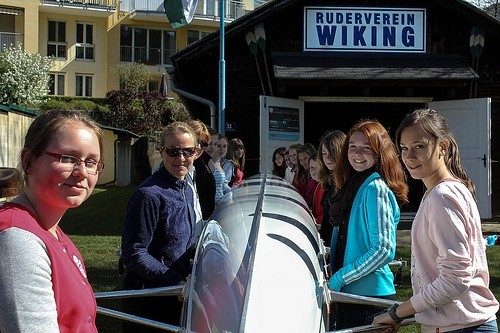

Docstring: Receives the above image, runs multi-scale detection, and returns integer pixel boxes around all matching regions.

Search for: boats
[94,168,416,333]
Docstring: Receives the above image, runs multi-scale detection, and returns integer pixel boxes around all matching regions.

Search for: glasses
[36,150,104,175]
[162,145,197,157]
[197,139,208,149]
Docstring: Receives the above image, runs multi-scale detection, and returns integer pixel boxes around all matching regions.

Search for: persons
[372,109,499,333]
[161,119,347,247]
[327,121,409,333]
[121,122,203,319]
[0,110,101,333]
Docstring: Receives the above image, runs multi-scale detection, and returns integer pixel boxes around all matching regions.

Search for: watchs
[388,304,405,323]
